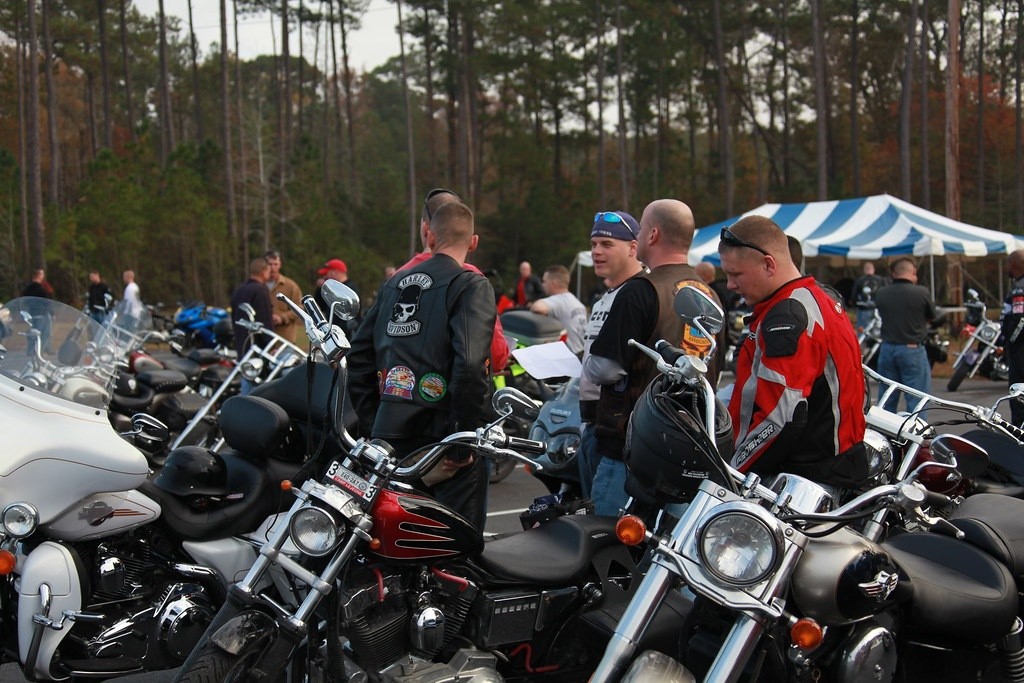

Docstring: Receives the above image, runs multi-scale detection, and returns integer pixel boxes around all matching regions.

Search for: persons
[718,216,865,502]
[123,271,140,342]
[22,269,56,355]
[579,199,726,519]
[391,189,509,372]
[347,203,497,535]
[852,257,935,422]
[229,249,396,396]
[88,271,112,340]
[512,261,579,355]
[999,250,1024,430]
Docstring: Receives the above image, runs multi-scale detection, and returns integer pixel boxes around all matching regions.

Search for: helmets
[626,374,733,503]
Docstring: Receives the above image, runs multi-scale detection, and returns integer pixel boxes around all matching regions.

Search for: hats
[591,211,638,240]
[319,259,347,275]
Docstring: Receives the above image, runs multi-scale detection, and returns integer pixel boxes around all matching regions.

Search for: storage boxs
[501,311,563,346]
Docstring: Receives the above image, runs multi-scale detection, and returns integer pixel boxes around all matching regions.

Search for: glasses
[721,227,769,256]
[424,188,461,221]
[594,212,636,241]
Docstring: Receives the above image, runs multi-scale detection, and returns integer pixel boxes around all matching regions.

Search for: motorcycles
[0,261,641,683]
[586,287,1024,683]
[946,288,1010,393]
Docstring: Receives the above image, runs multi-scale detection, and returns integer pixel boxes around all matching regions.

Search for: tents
[570,193,1024,308]
